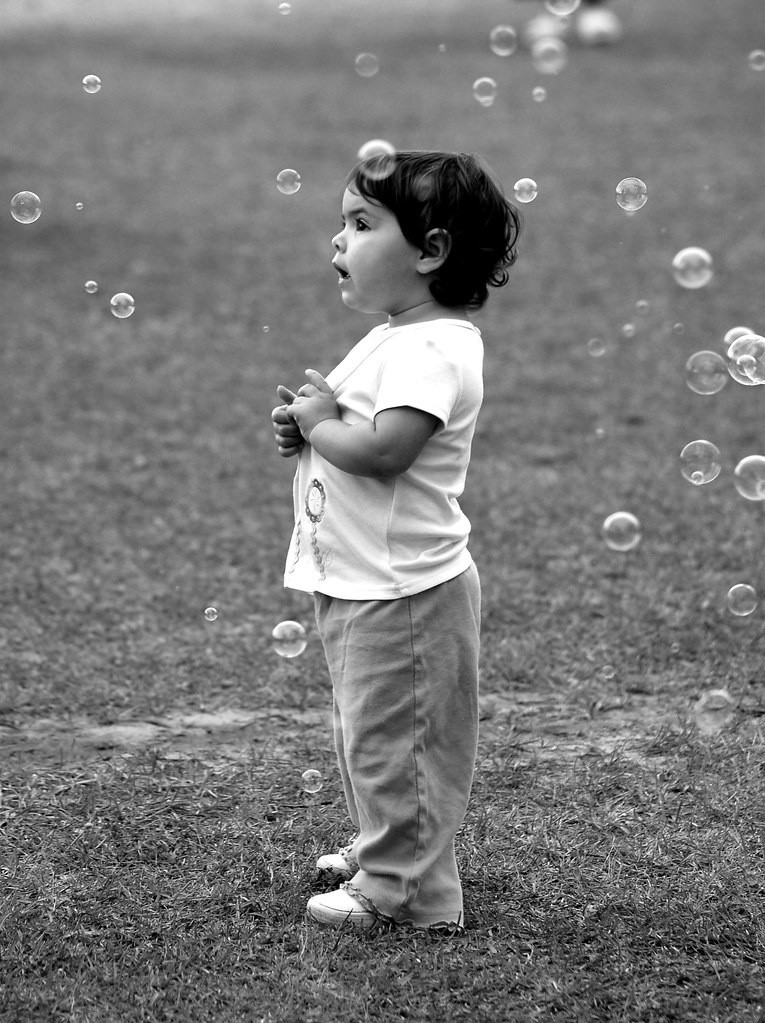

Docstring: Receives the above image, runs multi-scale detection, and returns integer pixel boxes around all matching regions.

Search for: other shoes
[317,853,356,885]
[306,885,388,933]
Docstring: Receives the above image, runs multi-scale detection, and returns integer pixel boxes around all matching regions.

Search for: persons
[270,151,519,931]
